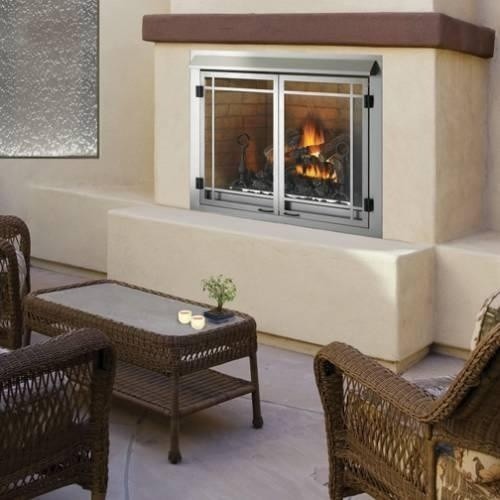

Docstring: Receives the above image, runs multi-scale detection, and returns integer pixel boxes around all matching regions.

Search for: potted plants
[201,274,237,323]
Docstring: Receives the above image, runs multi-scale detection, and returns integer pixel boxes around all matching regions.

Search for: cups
[178,310,192,324]
[191,314,206,330]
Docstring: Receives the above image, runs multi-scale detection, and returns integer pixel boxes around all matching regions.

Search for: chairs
[313,291,500,500]
[0,214,31,350]
[1,328,117,499]
